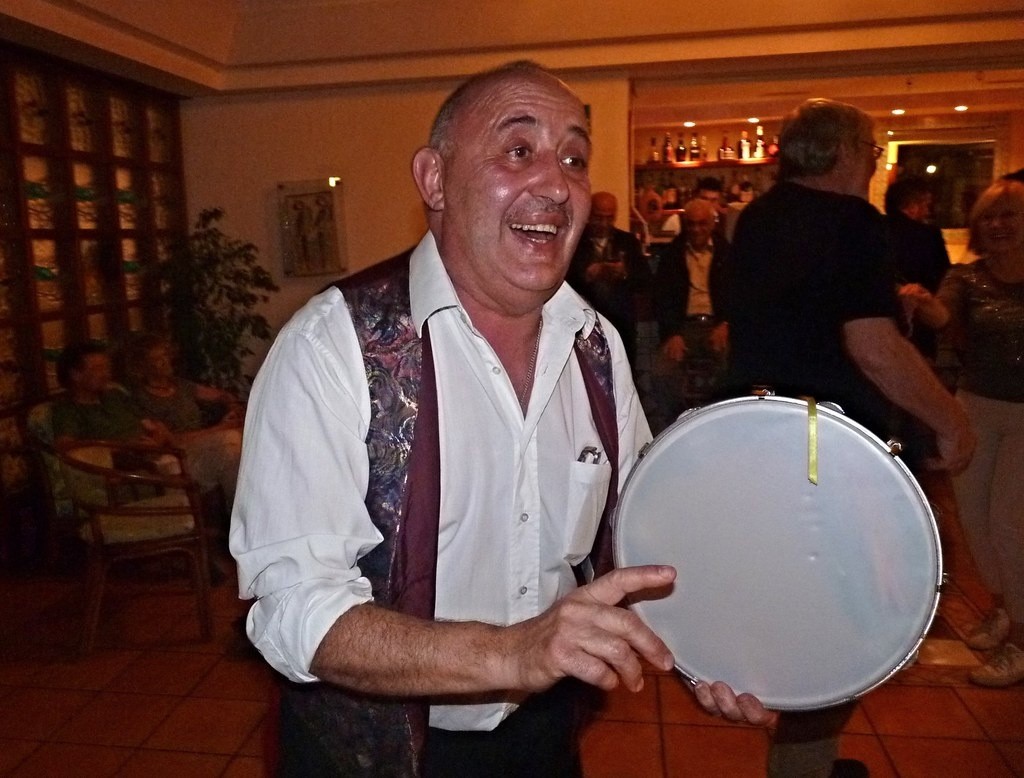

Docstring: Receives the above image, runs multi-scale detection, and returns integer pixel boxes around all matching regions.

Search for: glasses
[861,140,883,159]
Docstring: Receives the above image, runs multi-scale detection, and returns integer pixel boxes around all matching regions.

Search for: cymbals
[605,386,953,721]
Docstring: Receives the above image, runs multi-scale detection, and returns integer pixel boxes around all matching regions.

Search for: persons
[229,59,779,778]
[55,340,242,514]
[729,98,974,777]
[125,333,238,442]
[564,174,1023,688]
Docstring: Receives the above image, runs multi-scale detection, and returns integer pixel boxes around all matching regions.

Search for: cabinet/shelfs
[632,158,781,243]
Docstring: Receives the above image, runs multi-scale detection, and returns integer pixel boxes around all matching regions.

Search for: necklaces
[520,319,543,406]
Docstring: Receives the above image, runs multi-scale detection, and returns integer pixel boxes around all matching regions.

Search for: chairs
[58,438,212,655]
[17,393,161,596]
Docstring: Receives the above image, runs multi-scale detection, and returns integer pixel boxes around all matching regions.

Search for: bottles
[720,168,777,203]
[646,136,659,163]
[738,130,753,158]
[700,135,708,159]
[689,132,701,161]
[662,132,673,162]
[675,131,687,161]
[756,125,767,158]
[717,130,736,161]
[634,171,690,213]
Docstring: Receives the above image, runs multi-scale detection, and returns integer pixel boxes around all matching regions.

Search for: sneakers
[966,607,1012,651]
[969,642,1023,687]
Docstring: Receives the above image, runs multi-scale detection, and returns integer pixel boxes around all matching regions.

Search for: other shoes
[830,759,867,776]
